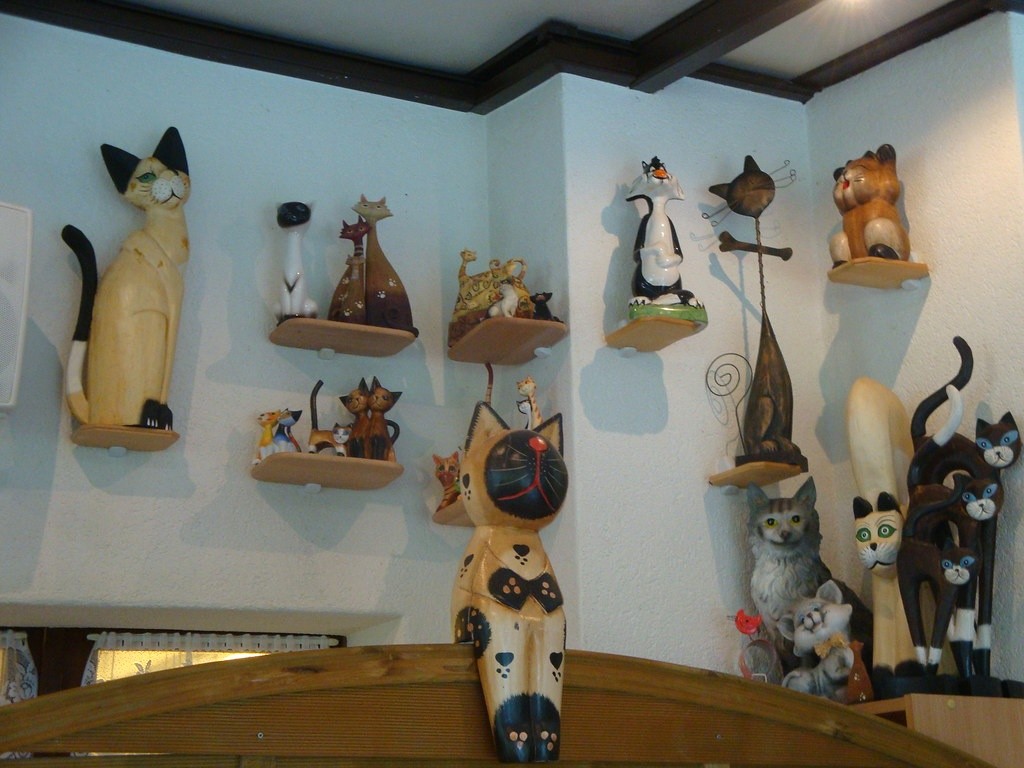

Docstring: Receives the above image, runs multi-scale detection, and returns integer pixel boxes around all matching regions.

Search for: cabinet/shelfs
[72,255,931,527]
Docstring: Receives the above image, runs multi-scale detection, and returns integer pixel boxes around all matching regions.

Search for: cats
[483,278,519,320]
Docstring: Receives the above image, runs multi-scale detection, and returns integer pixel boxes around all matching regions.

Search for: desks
[852,691,1024,767]
